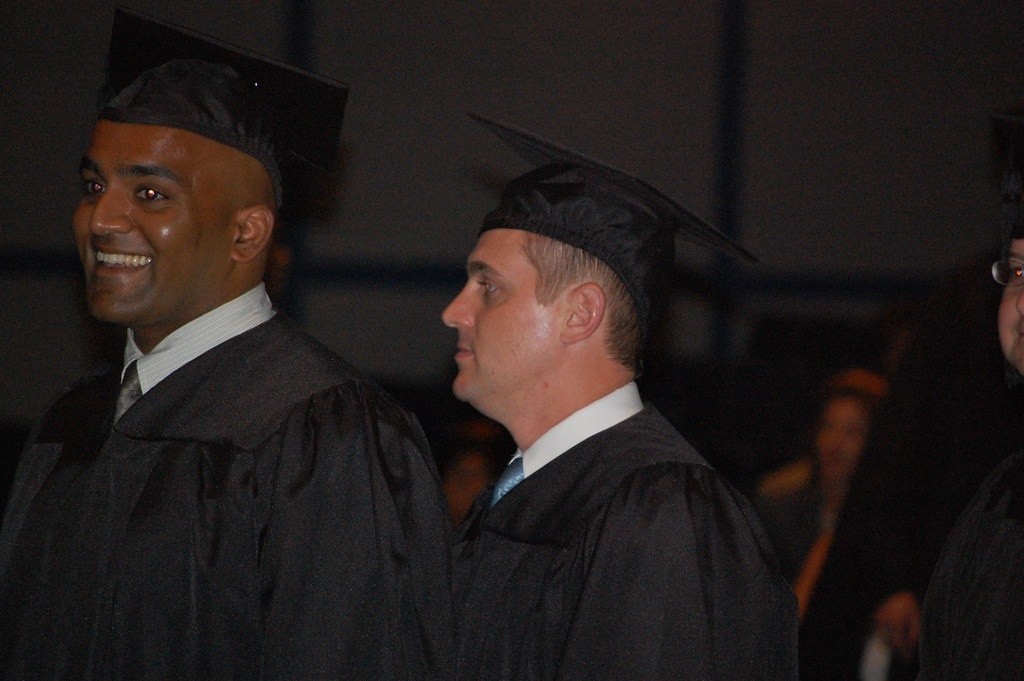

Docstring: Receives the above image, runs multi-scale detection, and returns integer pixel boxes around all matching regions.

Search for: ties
[108,359,143,436]
[488,457,523,508]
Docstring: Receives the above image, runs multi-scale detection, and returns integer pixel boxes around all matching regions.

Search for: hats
[992,113,1024,268]
[466,111,760,339]
[97,6,350,220]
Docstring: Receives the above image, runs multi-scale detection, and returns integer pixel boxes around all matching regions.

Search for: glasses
[992,260,1023,286]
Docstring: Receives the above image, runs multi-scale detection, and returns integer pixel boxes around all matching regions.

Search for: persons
[875,108,1024,681]
[752,364,888,674]
[0,4,456,680]
[442,112,799,680]
